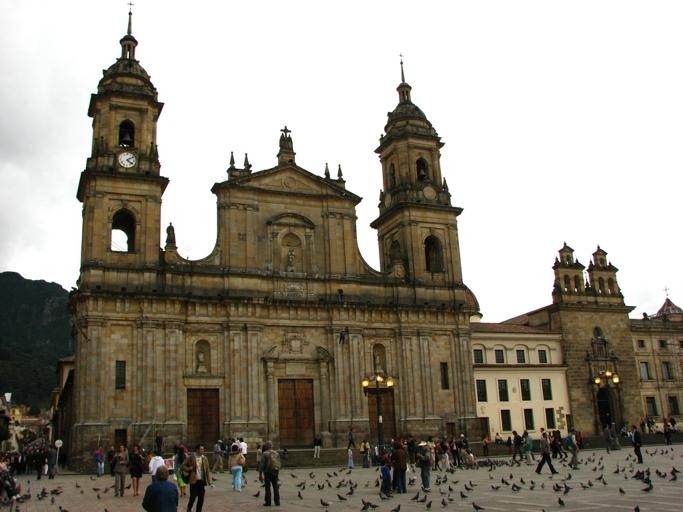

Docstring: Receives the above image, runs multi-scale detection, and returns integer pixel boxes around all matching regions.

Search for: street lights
[359,369,402,486]
[593,365,622,445]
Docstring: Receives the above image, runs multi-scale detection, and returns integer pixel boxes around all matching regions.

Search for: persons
[95,431,289,512]
[483,427,583,475]
[602,413,677,464]
[347,428,476,494]
[313,434,322,458]
[0,448,56,512]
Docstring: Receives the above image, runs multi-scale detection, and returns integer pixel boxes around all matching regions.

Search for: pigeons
[207,447,486,512]
[487,447,681,512]
[16,472,132,512]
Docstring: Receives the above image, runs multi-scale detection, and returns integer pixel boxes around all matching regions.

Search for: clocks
[116,149,136,170]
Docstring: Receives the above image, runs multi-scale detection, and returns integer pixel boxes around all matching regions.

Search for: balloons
[55,440,63,448]
